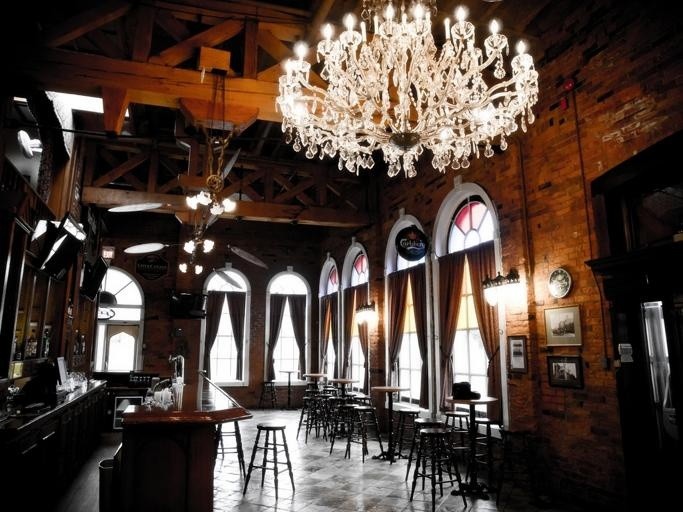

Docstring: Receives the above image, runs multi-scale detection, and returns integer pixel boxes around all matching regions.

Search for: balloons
[547,267,572,299]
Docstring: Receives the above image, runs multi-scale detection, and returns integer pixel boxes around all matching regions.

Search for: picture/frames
[279,371,301,410]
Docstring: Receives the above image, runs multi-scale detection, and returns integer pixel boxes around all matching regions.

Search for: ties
[37,212,88,283]
[54,357,67,385]
[81,255,109,301]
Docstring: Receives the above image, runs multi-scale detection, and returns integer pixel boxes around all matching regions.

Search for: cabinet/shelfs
[66,371,88,391]
[12,329,50,359]
[73,329,85,356]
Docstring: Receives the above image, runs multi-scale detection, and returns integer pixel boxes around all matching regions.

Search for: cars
[152,377,183,409]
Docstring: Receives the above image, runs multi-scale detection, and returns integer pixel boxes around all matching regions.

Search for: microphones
[215,421,247,479]
[242,424,296,499]
[295,372,509,511]
[258,382,279,410]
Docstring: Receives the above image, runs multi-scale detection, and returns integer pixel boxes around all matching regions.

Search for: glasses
[478,267,520,309]
[353,300,377,326]
[274,0,541,179]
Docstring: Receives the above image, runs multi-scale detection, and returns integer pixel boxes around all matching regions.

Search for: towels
[99,458,114,512]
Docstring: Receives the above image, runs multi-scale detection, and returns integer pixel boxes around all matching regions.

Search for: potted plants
[547,355,584,390]
[544,304,582,349]
[507,335,528,373]
